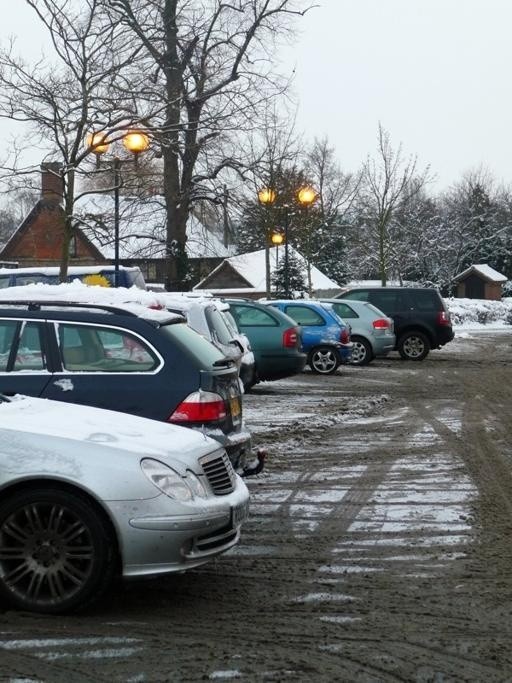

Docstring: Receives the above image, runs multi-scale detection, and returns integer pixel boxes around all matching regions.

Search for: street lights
[86,126,150,288]
[256,182,318,300]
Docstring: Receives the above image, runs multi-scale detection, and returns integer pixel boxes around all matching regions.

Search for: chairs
[57,344,85,368]
